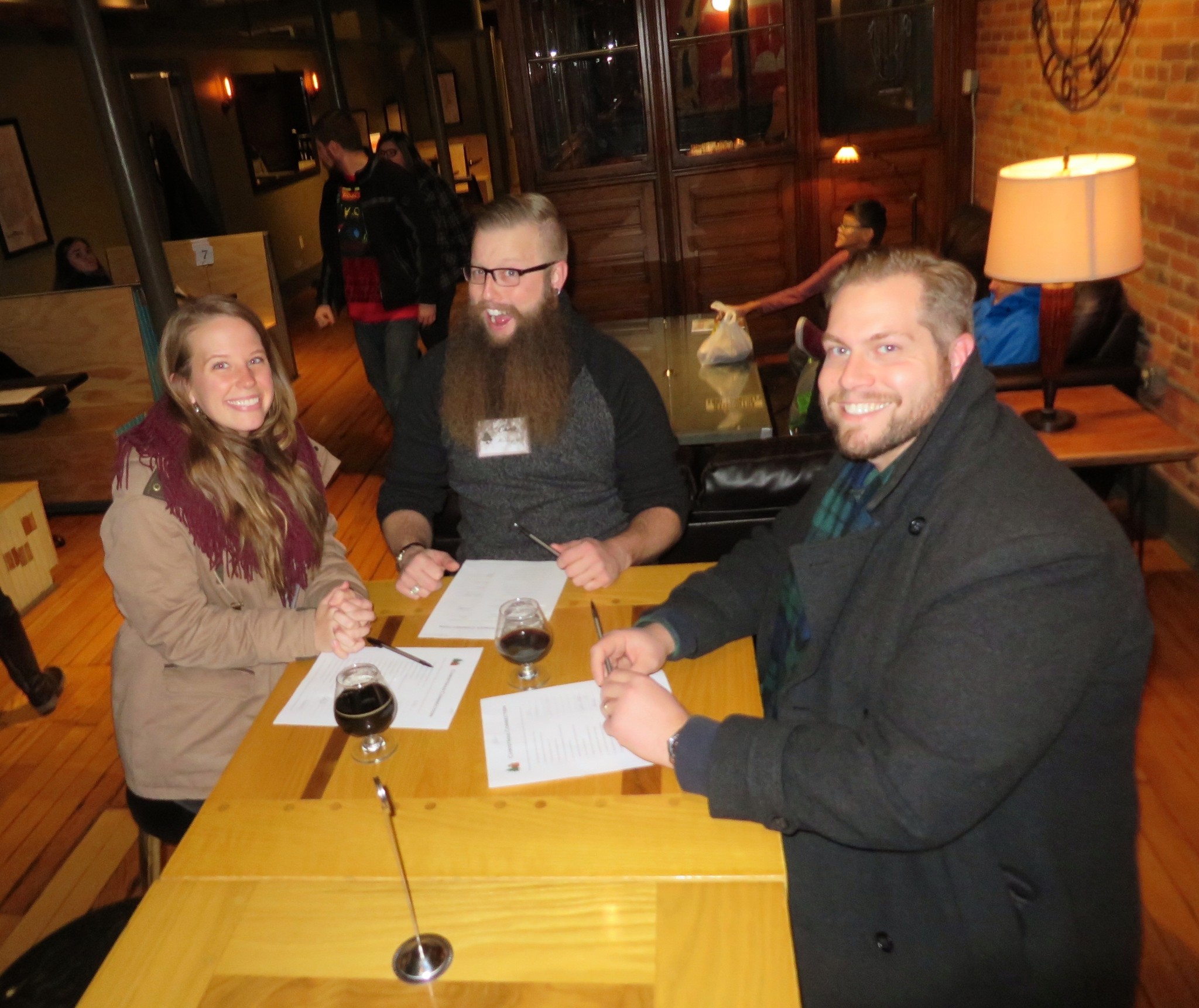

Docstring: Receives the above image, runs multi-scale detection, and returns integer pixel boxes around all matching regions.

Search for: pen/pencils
[589,600,613,676]
[365,635,434,668]
[514,522,560,557]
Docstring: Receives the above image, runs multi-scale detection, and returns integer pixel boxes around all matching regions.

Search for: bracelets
[394,542,428,563]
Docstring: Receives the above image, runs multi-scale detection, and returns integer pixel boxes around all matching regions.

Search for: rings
[603,698,612,716]
[409,586,421,596]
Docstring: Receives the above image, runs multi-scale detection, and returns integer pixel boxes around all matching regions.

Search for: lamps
[833,133,860,163]
[989,145,1147,433]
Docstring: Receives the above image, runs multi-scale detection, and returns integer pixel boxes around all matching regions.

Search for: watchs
[668,729,678,766]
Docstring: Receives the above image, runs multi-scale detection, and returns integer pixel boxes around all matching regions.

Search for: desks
[1,368,90,429]
[993,383,1198,573]
[82,555,809,1008]
[591,309,775,450]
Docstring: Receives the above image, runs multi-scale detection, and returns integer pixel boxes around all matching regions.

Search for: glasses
[839,221,863,232]
[380,148,399,158]
[462,260,556,287]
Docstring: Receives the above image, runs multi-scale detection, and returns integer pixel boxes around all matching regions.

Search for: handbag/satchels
[695,300,754,368]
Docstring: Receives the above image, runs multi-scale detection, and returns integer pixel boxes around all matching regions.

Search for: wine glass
[495,597,553,689]
[334,663,400,764]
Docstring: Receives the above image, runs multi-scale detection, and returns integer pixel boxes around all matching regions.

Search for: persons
[374,192,688,603]
[50,236,114,292]
[969,277,1043,367]
[713,194,889,360]
[309,109,439,421]
[374,129,468,349]
[96,291,377,847]
[592,249,1153,1008]
[0,586,64,730]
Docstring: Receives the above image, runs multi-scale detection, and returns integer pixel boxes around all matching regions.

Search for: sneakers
[795,316,827,362]
[35,664,65,717]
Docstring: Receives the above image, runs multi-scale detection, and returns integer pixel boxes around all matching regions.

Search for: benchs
[0,287,156,512]
[1,475,58,616]
[104,234,297,383]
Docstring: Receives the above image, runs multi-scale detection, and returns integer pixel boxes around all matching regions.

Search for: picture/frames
[0,119,56,258]
